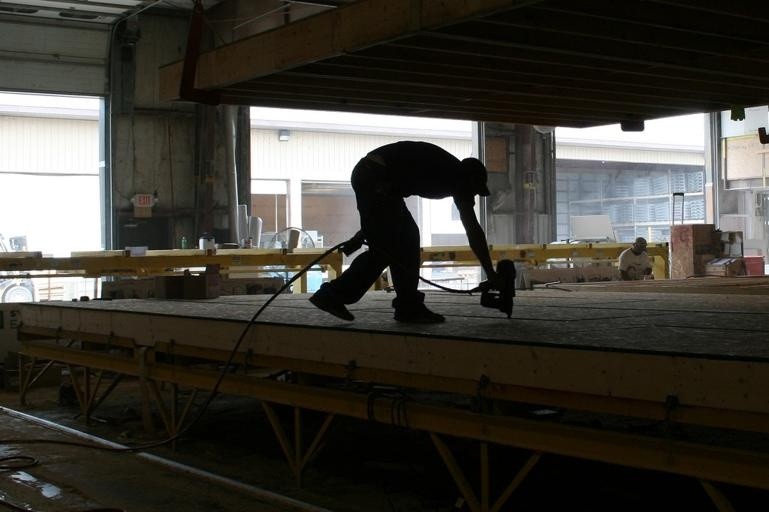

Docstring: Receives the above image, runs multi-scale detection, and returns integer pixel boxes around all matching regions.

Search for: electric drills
[480,259,516,318]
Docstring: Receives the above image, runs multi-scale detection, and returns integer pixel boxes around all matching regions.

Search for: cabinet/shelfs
[555,167,705,245]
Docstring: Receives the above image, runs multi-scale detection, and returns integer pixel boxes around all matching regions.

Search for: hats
[463,158,490,197]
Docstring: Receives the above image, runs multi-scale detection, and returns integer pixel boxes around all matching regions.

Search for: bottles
[181,236,188,249]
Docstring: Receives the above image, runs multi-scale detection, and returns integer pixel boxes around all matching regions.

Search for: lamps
[279,129,291,141]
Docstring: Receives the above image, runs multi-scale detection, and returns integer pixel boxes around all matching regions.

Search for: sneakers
[308,288,355,322]
[393,302,445,323]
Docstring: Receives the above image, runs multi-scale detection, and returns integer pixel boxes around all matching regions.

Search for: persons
[308,139,517,324]
[618,237,654,281]
